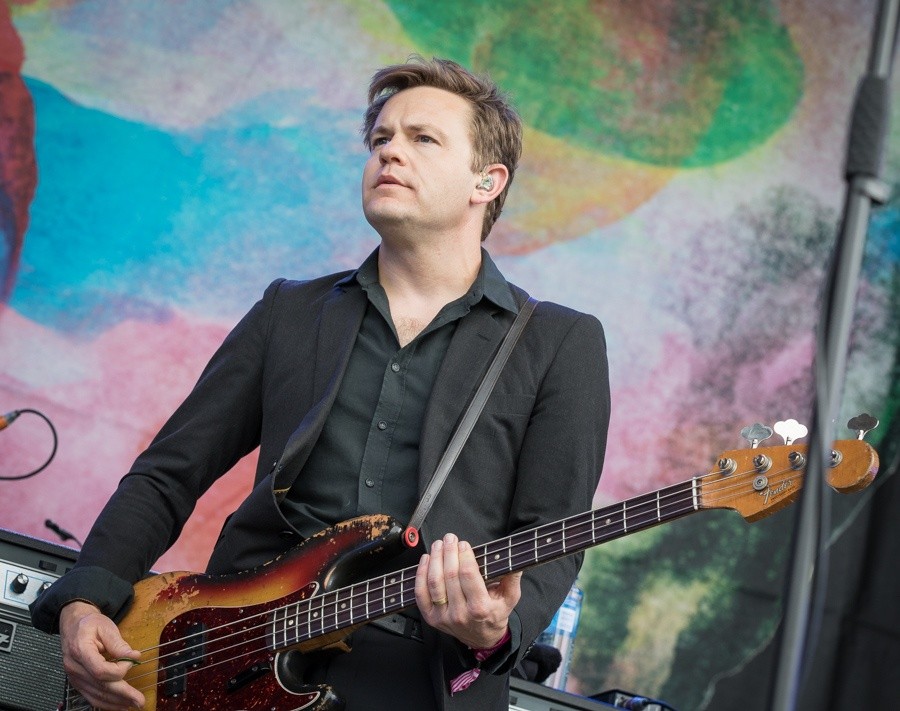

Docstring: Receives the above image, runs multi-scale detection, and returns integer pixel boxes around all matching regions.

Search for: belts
[371,612,427,644]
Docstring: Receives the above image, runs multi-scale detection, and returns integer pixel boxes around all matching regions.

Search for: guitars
[99,413,880,710]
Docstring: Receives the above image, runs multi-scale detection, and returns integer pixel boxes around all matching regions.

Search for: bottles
[533,576,584,691]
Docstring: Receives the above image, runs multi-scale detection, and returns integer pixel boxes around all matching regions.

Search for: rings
[433,597,447,606]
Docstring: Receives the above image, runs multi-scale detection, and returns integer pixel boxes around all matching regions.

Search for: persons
[29,54,611,711]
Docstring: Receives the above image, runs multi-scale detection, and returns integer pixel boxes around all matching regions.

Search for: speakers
[1,526,159,711]
